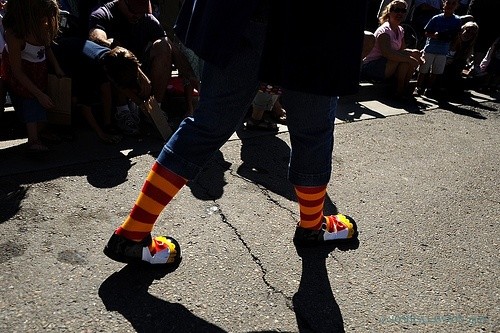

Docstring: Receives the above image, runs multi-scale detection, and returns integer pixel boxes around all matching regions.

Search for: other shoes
[101,229,181,267]
[292,213,359,246]
[103,123,123,137]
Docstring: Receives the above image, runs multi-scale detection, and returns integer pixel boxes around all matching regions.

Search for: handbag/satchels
[47,73,72,126]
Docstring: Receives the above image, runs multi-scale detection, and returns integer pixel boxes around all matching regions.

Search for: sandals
[246,116,278,132]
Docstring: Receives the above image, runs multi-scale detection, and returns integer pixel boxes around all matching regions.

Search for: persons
[0,0,75,152]
[75,0,202,145]
[367,0,423,102]
[246,85,288,134]
[103,0,365,268]
[418,0,462,96]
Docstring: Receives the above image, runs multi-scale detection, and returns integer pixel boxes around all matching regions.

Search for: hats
[123,0,149,15]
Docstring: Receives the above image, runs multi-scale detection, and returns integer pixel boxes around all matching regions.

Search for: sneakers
[114,109,139,135]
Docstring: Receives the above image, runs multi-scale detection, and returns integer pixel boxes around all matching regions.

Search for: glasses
[391,7,407,14]
